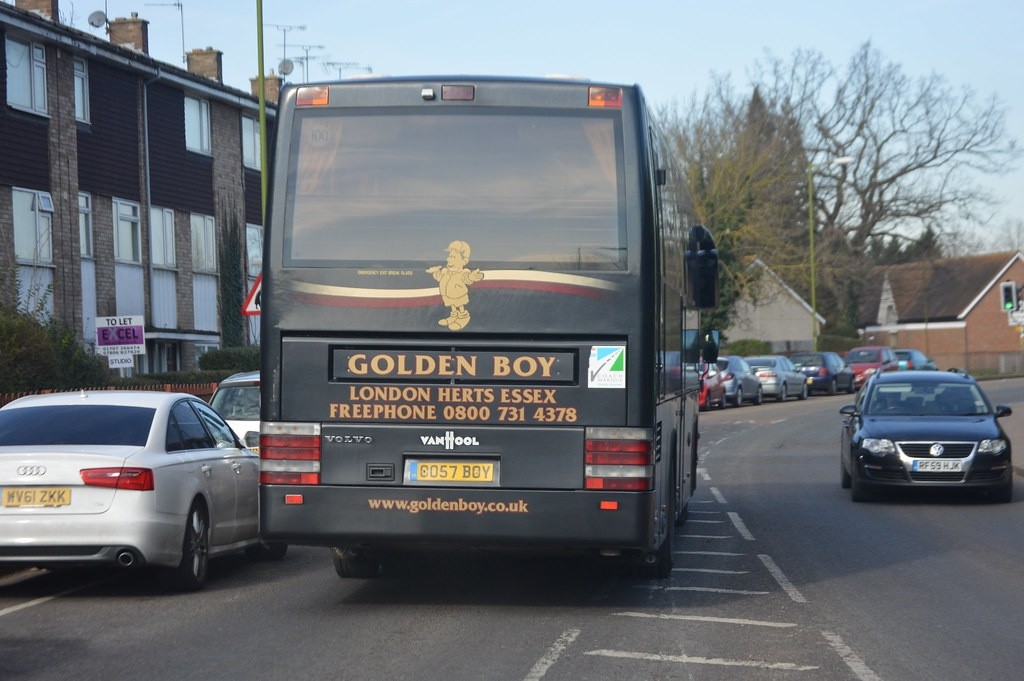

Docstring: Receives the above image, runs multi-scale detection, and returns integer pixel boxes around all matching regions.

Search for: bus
[255,75,720,583]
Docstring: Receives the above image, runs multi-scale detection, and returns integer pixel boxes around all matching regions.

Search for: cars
[744,355,810,402]
[788,351,856,396]
[895,349,939,370]
[208,369,261,456]
[698,363,734,410]
[837,365,1014,503]
[1,390,288,594]
[716,353,763,407]
[843,346,901,391]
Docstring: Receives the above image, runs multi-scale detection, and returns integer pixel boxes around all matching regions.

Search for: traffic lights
[999,282,1017,311]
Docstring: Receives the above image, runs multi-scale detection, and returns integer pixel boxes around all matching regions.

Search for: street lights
[266,22,361,82]
[806,156,858,350]
